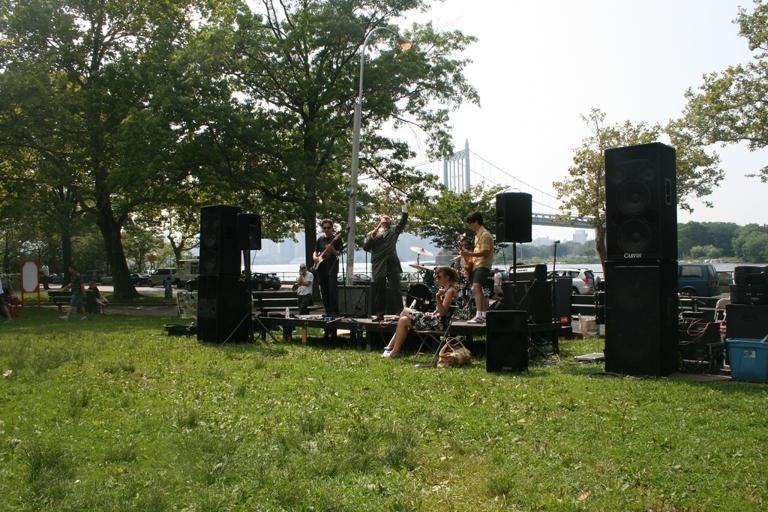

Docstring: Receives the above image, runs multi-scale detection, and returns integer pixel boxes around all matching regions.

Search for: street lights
[345,26,412,286]
[553,240,561,271]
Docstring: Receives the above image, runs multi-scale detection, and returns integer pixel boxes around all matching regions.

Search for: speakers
[496,193,532,243]
[605,143,678,261]
[236,214,262,250]
[486,310,528,372]
[200,205,240,276]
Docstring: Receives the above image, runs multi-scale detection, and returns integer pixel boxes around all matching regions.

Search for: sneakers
[372,315,384,323]
[466,315,484,324]
[58,315,88,321]
[481,317,487,323]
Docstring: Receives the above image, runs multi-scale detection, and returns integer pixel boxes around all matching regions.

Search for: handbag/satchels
[436,335,474,369]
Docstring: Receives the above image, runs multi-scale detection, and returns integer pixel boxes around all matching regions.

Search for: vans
[677,264,720,296]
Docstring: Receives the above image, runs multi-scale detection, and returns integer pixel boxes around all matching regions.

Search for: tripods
[479,242,547,360]
[221,250,280,345]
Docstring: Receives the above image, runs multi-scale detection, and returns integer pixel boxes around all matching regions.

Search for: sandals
[380,350,402,359]
[384,344,395,351]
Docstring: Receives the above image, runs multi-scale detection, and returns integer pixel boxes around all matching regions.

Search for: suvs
[547,267,602,296]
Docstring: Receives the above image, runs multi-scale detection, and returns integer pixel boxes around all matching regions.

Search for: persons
[465,211,495,324]
[457,240,474,275]
[382,266,458,359]
[297,264,315,317]
[312,219,345,317]
[0,260,111,326]
[491,267,504,295]
[362,195,410,321]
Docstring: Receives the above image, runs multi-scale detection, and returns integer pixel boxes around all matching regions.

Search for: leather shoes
[322,313,332,317]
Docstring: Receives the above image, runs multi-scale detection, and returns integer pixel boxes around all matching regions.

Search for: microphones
[373,223,381,237]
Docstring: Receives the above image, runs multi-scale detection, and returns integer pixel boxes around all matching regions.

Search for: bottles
[284,307,291,319]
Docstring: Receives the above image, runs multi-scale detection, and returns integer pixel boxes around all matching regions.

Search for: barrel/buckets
[576,315,599,339]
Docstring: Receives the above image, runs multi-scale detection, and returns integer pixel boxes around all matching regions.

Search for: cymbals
[410,264,428,270]
[410,246,433,257]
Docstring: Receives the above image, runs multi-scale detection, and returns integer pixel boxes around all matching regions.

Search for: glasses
[323,227,333,229]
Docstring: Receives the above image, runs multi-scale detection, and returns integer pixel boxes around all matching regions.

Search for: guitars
[313,233,341,271]
[458,245,474,276]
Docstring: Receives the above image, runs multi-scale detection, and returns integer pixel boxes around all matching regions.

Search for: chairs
[410,303,459,369]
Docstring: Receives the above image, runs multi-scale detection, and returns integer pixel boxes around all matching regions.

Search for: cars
[49,259,200,291]
[336,273,373,284]
[251,272,282,290]
[386,272,424,291]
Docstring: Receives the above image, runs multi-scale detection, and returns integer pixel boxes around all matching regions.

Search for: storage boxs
[725,338,768,383]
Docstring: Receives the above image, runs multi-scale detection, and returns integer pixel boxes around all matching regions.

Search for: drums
[423,269,434,285]
[406,284,435,311]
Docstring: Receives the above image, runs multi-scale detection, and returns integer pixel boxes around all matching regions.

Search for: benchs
[250,290,324,340]
[48,291,105,314]
